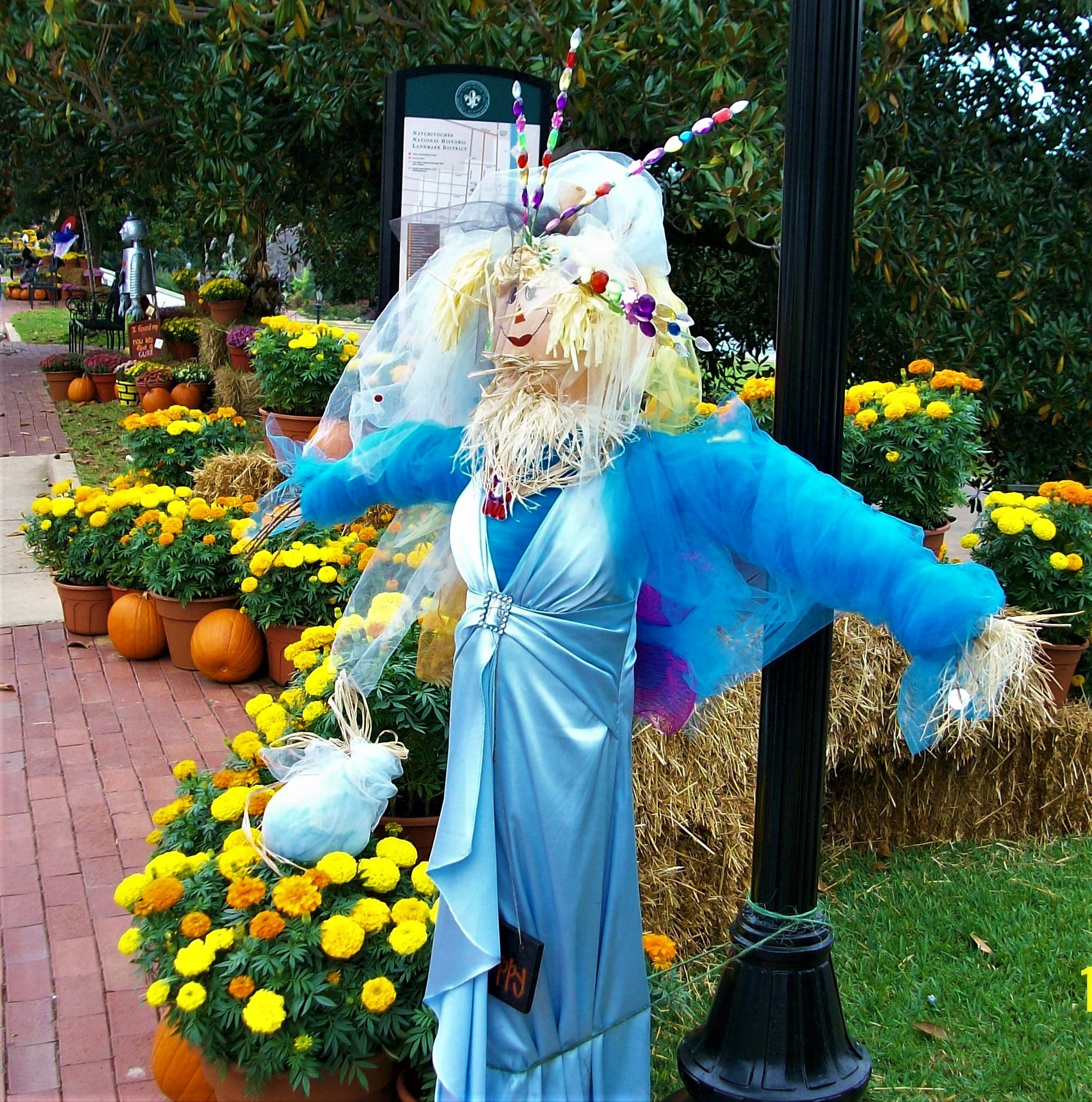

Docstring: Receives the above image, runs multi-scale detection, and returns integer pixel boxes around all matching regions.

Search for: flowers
[0,229,105,293]
[6,268,1092,1096]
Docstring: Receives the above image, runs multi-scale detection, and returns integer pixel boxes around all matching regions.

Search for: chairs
[65,271,125,355]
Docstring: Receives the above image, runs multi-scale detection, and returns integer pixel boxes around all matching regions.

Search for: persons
[251,27,1008,1102]
[119,210,157,323]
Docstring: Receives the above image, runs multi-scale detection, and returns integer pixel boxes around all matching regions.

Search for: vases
[1036,626,1088,707]
[923,514,948,559]
[3,257,434,1101]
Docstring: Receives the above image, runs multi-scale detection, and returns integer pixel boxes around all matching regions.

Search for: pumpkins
[6,287,46,301]
[152,1009,219,1102]
[107,593,167,661]
[170,380,201,409]
[309,420,353,459]
[142,381,173,412]
[188,608,264,682]
[68,372,96,401]
